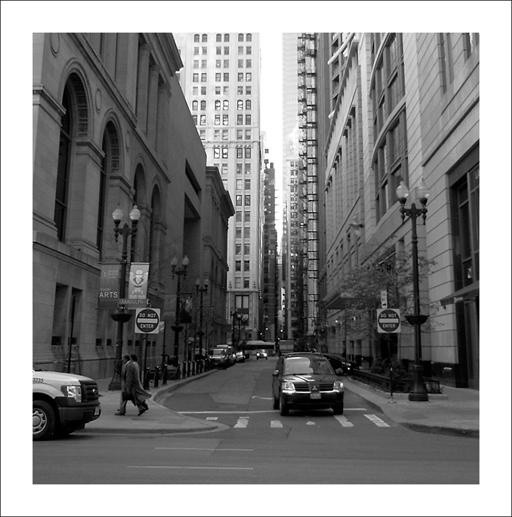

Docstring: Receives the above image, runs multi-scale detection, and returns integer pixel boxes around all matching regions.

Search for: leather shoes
[114,409,126,416]
[138,405,148,416]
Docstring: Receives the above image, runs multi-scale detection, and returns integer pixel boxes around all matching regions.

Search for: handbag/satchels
[122,387,133,400]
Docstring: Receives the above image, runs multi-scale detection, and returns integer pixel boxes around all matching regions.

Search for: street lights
[109,205,140,390]
[236,311,241,340]
[171,256,189,358]
[195,278,208,358]
[396,178,430,402]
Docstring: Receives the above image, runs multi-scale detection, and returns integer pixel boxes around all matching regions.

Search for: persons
[114,353,147,416]
[117,353,149,411]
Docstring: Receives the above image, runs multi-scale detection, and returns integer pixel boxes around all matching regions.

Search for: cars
[207,344,249,369]
[161,358,183,380]
[272,352,345,417]
[256,349,268,361]
[317,352,359,375]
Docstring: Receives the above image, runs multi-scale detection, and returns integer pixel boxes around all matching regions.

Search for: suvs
[33,369,102,441]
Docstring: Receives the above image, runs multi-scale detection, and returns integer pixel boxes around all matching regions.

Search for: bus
[244,340,277,356]
